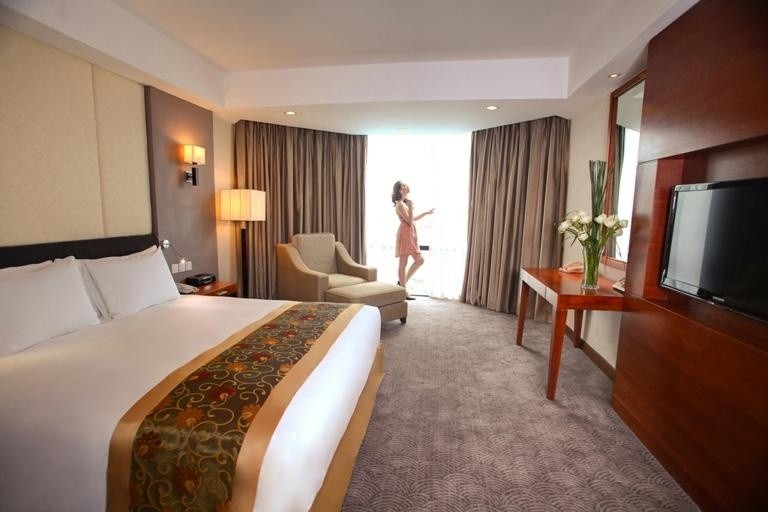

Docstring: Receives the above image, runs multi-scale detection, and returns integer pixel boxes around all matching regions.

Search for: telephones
[175,282,199,294]
[612,277,625,293]
[558,260,583,273]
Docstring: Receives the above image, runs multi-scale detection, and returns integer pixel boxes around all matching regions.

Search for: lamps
[181,143,206,186]
[162,239,186,264]
[219,189,267,298]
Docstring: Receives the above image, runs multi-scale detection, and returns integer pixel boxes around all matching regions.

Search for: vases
[576,245,605,288]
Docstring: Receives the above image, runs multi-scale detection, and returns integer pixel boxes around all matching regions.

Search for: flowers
[557,156,628,288]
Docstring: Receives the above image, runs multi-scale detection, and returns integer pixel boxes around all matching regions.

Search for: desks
[514,263,625,403]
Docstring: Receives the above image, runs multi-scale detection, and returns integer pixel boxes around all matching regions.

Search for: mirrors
[600,66,648,272]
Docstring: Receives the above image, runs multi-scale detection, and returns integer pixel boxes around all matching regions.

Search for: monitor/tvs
[659,176,768,324]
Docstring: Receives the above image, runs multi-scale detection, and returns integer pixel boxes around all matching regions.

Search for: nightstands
[180,279,240,296]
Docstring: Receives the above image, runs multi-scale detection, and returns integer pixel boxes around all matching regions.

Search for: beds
[0,233,384,510]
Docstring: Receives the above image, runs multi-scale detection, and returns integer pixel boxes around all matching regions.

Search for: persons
[392,181,434,300]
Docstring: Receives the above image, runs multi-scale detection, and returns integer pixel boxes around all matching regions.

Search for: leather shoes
[407,297,414,300]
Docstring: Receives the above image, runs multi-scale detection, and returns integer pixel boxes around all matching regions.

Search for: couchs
[276,231,407,329]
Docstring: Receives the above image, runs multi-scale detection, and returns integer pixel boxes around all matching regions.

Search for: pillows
[0,255,100,358]
[75,245,158,324]
[86,245,180,318]
